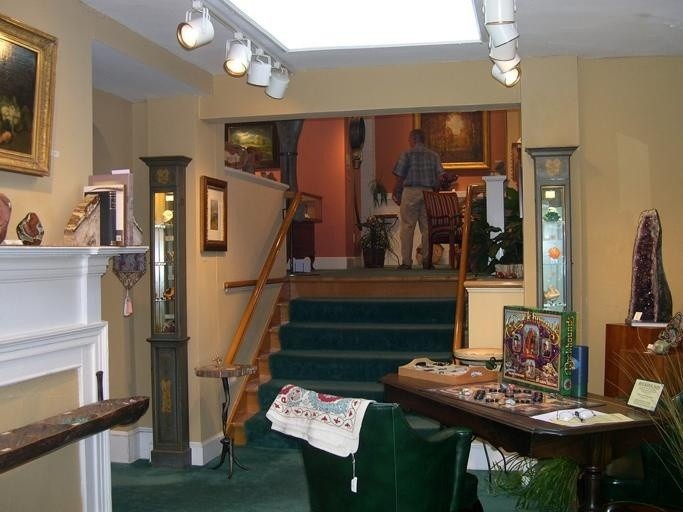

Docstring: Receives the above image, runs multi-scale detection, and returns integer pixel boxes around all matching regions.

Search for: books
[82,168,133,247]
[625,319,668,327]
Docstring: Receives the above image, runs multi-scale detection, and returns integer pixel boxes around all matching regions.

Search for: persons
[391,129,447,270]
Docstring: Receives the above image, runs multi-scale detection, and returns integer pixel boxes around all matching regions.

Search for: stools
[451,348,507,484]
[194,362,257,479]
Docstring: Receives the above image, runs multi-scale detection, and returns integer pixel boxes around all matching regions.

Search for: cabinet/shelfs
[285,190,324,270]
[525,142,578,313]
[136,155,191,470]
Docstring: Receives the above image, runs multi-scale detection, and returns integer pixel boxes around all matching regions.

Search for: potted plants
[488,221,523,278]
[354,213,400,266]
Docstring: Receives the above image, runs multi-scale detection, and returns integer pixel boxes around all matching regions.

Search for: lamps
[174,1,289,100]
[481,2,521,88]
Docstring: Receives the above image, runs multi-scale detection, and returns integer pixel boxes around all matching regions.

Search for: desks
[379,357,682,512]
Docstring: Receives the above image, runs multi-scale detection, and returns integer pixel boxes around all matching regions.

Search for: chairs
[290,402,484,511]
[421,190,464,270]
[579,390,682,511]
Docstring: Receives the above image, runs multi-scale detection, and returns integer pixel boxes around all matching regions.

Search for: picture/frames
[198,174,227,251]
[224,120,280,169]
[411,111,492,173]
[0,12,60,181]
